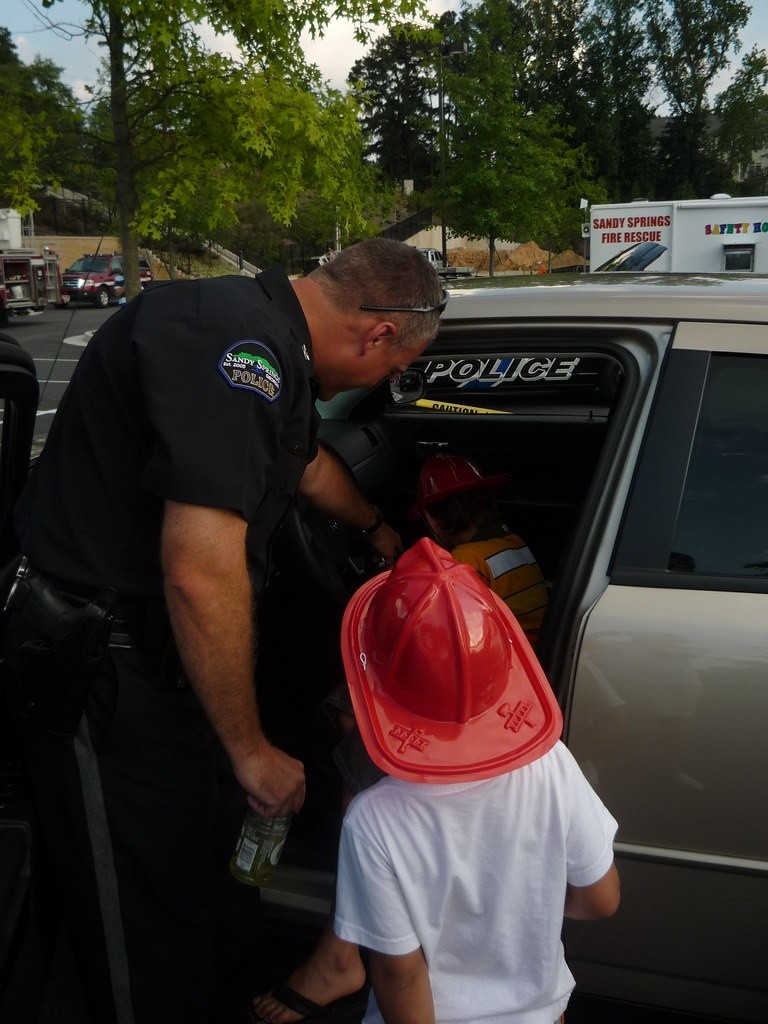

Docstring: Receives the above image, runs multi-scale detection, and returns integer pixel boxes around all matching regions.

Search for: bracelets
[359,506,384,535]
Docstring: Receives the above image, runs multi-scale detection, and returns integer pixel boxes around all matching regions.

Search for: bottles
[229,807,293,887]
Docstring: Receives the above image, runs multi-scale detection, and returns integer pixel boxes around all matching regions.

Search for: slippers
[248,974,371,1024]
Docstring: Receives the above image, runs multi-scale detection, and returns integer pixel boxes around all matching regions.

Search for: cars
[0,265,767,1024]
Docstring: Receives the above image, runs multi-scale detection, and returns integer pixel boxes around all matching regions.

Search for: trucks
[585,190,768,273]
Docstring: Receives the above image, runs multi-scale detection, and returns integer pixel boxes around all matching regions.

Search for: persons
[252,453,549,1024]
[0,238,443,1024]
[334,536,621,1024]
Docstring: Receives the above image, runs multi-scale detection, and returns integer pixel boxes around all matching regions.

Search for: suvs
[417,248,451,271]
[55,254,155,311]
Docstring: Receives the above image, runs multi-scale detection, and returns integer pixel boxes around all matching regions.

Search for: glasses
[357,289,451,314]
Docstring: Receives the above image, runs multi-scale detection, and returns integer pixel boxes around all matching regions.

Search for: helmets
[339,537,565,785]
[407,453,509,519]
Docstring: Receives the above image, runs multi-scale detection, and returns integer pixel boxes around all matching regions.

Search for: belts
[115,610,174,646]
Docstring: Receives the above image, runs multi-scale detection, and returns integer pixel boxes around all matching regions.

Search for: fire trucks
[0,206,64,326]
